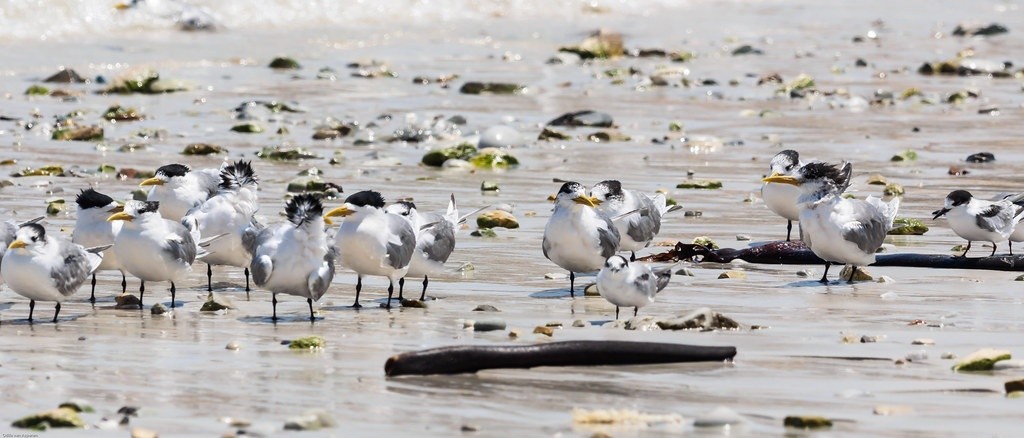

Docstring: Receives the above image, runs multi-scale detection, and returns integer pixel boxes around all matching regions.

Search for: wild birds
[760,151,1024,285]
[0,159,257,322]
[243,191,493,321]
[541,179,671,320]
